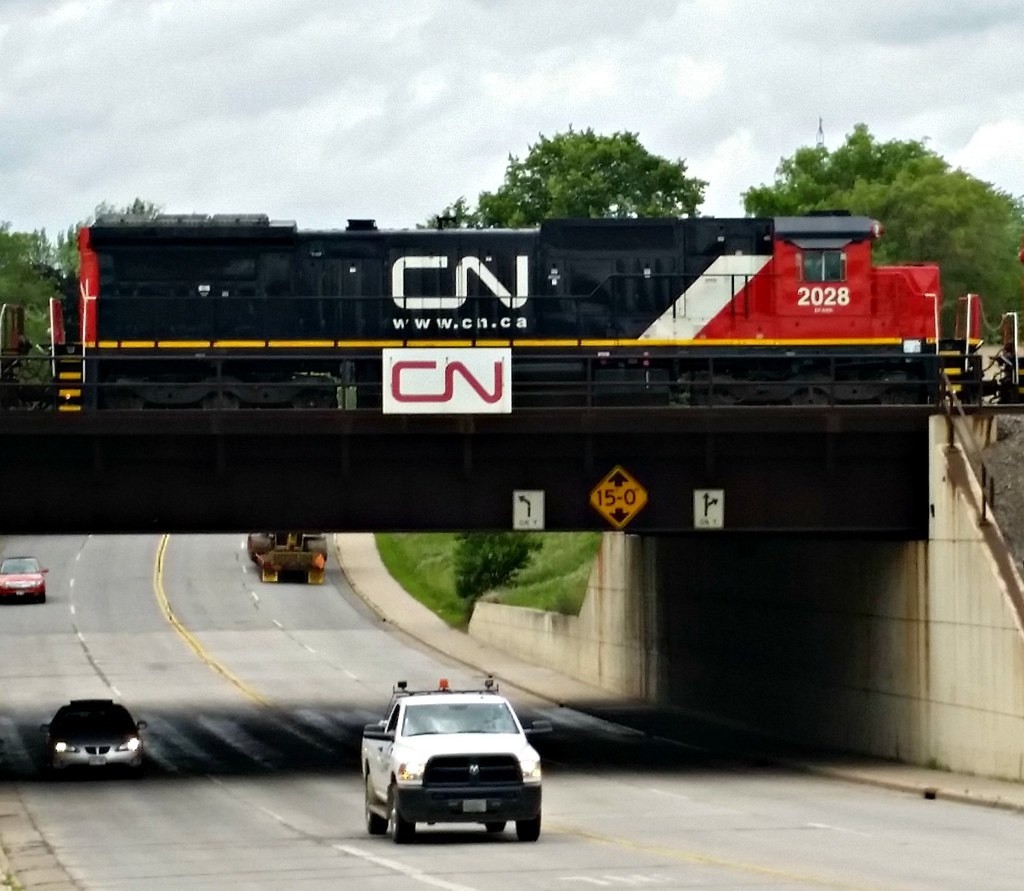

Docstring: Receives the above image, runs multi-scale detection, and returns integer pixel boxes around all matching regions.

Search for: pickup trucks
[360,679,543,840]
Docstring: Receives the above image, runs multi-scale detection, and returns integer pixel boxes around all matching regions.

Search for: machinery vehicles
[248,532,328,586]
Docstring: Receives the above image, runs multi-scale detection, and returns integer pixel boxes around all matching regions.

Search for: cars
[40,701,148,775]
[0,555,49,606]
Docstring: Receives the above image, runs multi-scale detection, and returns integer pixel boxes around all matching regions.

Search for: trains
[0,216,1024,406]
